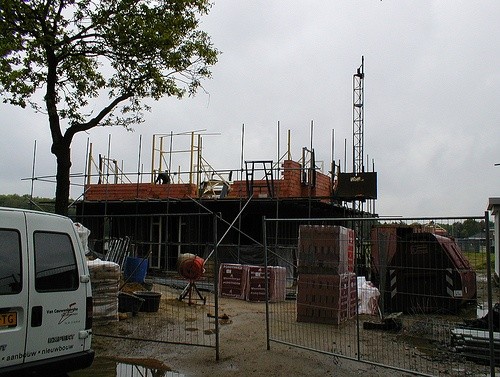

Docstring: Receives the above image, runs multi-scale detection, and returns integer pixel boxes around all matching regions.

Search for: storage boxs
[246,266,286,302]
[217,263,249,300]
[296,225,358,328]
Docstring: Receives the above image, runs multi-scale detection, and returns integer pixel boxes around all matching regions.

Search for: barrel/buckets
[133,290,162,312]
[118,291,145,314]
[124,256,148,282]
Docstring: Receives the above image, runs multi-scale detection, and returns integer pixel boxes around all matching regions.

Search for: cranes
[351,55,364,200]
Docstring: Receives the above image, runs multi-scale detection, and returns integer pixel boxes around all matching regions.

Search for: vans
[0,205,95,377]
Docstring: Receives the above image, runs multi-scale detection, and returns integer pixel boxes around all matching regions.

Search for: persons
[155,173,171,184]
